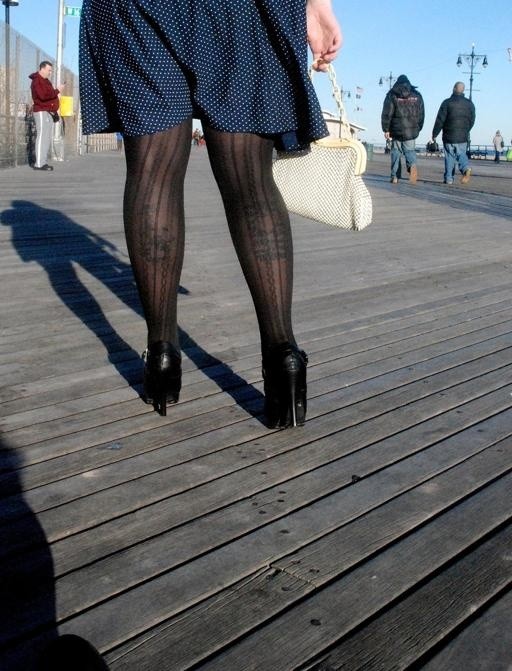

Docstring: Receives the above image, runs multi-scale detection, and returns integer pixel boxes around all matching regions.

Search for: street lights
[379,71,398,94]
[455,42,488,102]
[332,84,353,129]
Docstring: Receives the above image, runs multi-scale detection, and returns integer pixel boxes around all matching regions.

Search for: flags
[356,86,363,93]
[356,94,362,99]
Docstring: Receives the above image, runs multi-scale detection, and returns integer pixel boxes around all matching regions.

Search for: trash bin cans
[363,143,374,162]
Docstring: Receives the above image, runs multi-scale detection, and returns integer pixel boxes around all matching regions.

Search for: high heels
[261,343,309,431]
[142,340,182,417]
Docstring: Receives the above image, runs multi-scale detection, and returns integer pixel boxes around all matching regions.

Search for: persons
[432,82,475,184]
[29,62,66,171]
[386,139,392,155]
[493,130,503,163]
[193,128,201,147]
[381,74,425,183]
[116,132,122,151]
[79,0,342,428]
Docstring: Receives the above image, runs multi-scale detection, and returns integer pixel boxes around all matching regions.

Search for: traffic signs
[63,5,82,17]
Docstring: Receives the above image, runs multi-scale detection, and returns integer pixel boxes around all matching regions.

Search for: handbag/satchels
[501,142,504,148]
[263,57,373,231]
[46,110,60,123]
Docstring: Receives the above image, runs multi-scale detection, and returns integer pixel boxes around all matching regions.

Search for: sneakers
[33,164,54,171]
[444,168,472,184]
[391,163,417,184]
